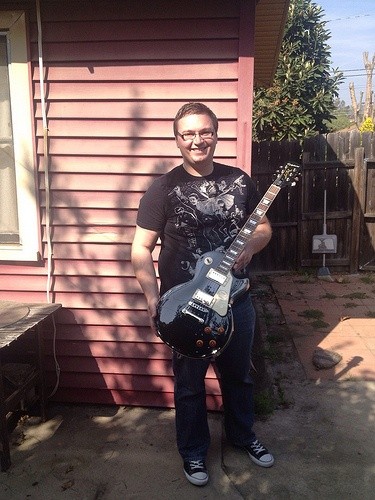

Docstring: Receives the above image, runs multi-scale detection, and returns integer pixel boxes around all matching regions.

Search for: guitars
[152,162,303,360]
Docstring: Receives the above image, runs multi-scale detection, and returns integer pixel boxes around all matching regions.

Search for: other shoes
[184,459,209,486]
[244,439,274,467]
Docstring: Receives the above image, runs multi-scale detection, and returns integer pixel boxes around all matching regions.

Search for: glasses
[177,131,213,140]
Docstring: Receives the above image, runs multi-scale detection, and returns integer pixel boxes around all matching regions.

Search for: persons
[131,103,274,485]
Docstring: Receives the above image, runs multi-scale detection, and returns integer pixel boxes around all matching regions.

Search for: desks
[0,303,62,471]
